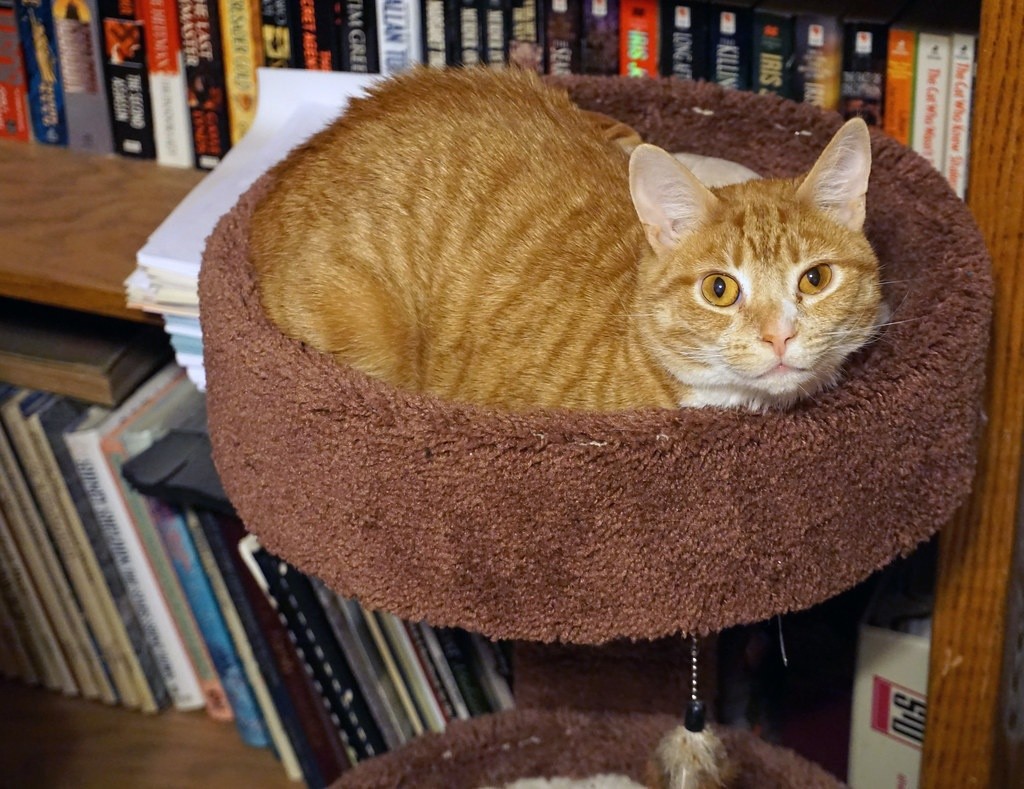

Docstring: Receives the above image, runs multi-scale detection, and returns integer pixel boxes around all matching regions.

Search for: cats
[241,58,928,416]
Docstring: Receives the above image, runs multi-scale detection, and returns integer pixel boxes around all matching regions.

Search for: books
[0,297,931,789]
[0,0,977,203]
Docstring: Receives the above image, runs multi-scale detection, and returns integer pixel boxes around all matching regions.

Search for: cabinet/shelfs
[0,0,1024,789]
